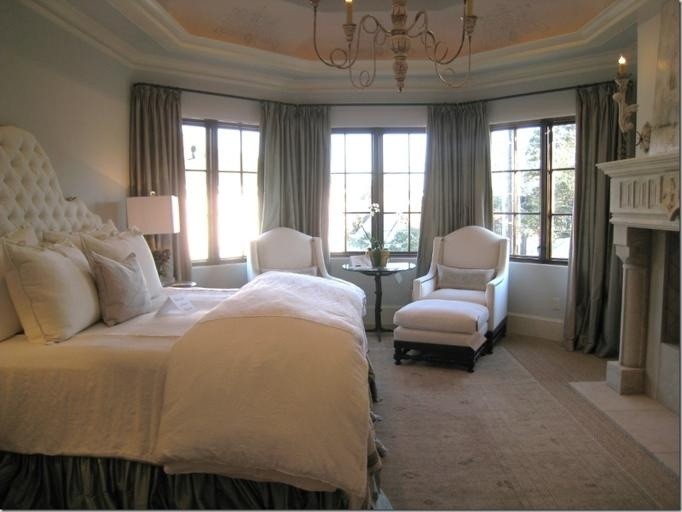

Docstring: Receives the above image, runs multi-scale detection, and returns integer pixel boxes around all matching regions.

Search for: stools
[392,298,489,372]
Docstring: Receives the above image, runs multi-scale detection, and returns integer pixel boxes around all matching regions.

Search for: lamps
[611,54,651,152]
[310,0,479,92]
[126,191,180,252]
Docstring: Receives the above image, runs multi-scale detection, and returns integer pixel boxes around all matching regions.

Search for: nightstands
[168,280,195,287]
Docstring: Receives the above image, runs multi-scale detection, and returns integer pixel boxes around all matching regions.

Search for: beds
[0,124,371,510]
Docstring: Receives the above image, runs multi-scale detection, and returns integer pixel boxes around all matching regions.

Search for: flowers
[350,202,406,250]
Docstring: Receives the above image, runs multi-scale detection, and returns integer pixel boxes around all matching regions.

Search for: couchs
[411,225,510,354]
[247,226,356,286]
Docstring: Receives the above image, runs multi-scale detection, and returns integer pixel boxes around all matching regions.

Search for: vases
[366,246,390,268]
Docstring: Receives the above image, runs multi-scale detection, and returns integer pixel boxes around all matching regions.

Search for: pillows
[436,265,497,289]
[259,267,319,276]
[0,220,163,348]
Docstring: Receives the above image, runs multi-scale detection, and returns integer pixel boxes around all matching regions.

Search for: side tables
[342,262,416,341]
